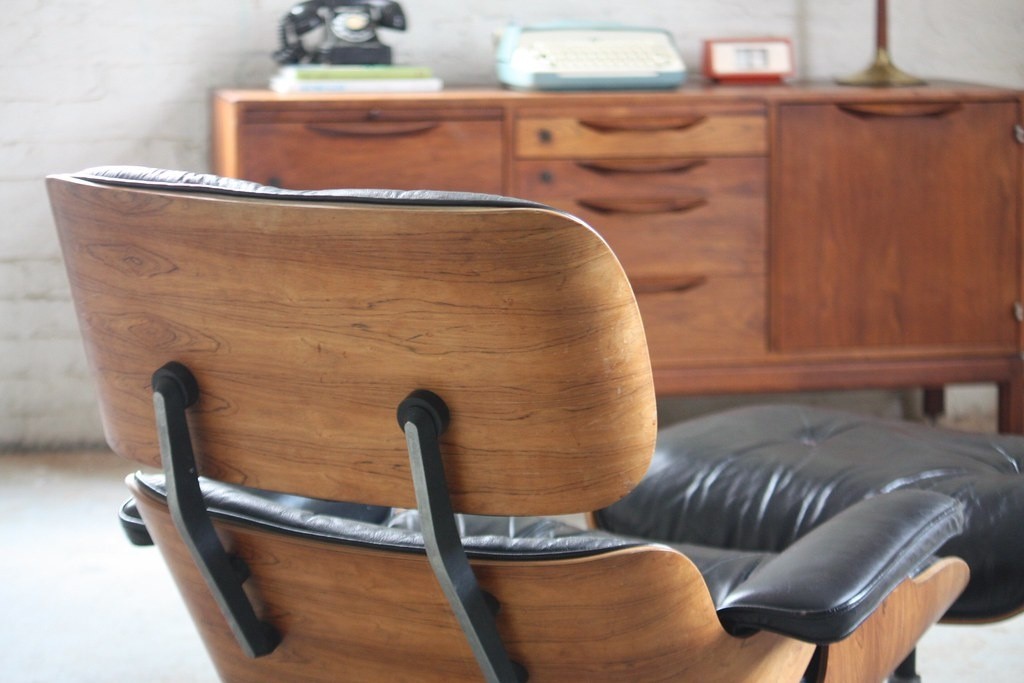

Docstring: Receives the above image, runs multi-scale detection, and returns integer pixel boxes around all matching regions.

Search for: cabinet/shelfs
[212,77,1024,435]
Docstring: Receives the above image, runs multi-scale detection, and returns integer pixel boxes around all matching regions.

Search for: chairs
[45,164,969,683]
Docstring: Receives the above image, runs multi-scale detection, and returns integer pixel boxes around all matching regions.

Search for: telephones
[279,0,408,64]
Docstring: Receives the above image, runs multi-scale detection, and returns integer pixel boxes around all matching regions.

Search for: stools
[593,404,1023,624]
